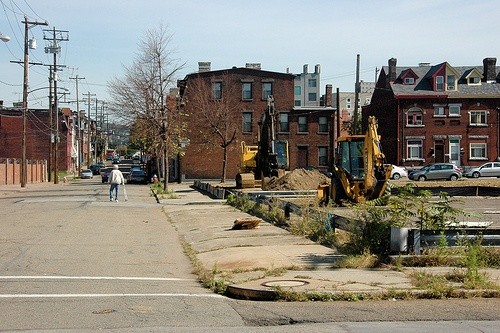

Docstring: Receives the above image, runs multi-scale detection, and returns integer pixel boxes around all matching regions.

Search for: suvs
[408,163,462,182]
[89,165,101,175]
[100,167,117,184]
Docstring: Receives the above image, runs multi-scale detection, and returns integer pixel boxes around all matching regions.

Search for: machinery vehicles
[331,115,393,205]
[236,94,290,189]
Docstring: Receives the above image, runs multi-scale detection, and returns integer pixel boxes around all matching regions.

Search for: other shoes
[110,198,113,201]
[115,199,118,203]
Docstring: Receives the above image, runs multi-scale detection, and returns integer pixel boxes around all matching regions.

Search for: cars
[112,155,146,185]
[382,163,409,181]
[80,169,93,179]
[465,162,500,178]
[98,162,105,168]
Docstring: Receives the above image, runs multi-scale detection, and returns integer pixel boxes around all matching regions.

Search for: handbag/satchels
[123,185,128,201]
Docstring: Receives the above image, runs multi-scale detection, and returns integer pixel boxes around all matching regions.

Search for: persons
[108,164,124,202]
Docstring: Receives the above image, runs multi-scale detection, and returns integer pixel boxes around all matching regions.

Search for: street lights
[20,86,69,187]
